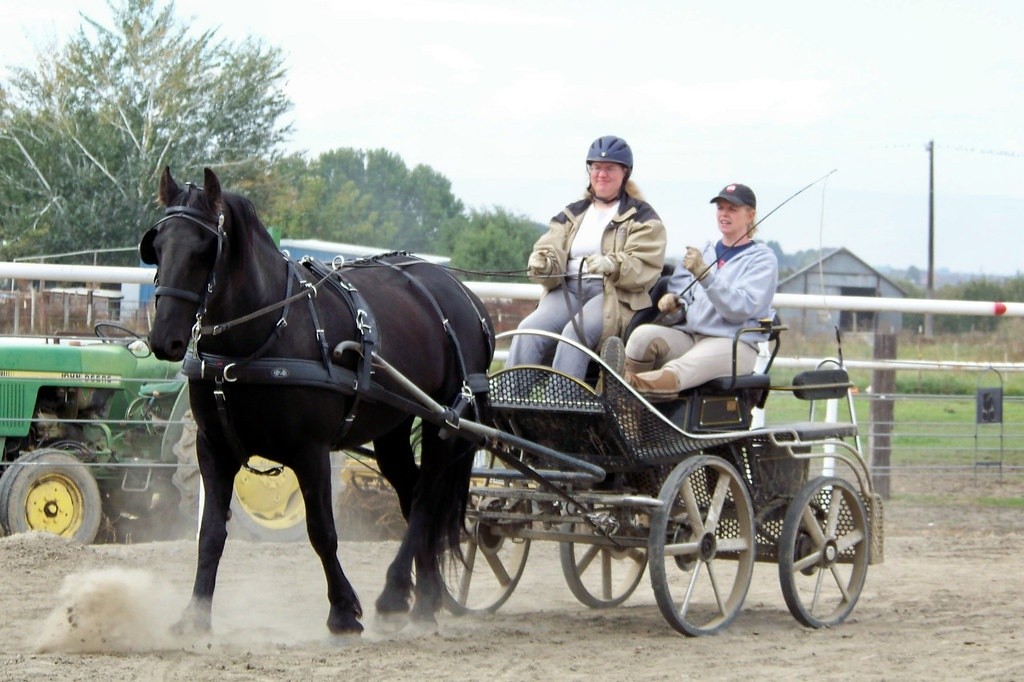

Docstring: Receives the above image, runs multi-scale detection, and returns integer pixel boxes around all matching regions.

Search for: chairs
[537,264,788,408]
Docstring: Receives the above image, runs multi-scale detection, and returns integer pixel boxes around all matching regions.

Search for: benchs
[757,358,860,441]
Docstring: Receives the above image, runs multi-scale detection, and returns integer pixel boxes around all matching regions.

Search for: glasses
[587,164,626,173]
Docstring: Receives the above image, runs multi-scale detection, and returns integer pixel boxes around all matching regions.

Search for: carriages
[139,163,873,647]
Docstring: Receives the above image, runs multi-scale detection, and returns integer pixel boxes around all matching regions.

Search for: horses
[138,164,496,647]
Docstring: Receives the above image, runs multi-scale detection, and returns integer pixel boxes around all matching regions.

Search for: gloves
[584,255,615,273]
[528,251,547,272]
[657,292,679,312]
[682,247,709,281]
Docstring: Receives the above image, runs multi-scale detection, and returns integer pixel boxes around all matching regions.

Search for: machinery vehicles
[1,235,545,544]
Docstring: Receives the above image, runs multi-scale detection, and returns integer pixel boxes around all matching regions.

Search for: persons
[499,136,667,400]
[601,183,779,444]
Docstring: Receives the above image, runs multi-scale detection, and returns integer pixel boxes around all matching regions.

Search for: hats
[710,183,756,208]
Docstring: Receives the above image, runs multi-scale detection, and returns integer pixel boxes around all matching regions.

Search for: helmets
[586,137,633,173]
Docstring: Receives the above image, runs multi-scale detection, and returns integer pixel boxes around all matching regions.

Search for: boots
[599,336,678,409]
[624,356,655,373]
